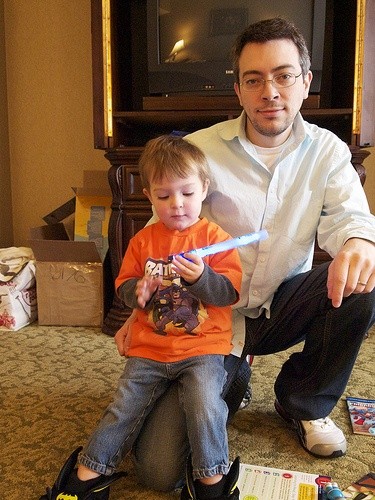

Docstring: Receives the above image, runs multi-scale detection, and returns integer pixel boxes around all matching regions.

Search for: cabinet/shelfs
[104,145,370,333]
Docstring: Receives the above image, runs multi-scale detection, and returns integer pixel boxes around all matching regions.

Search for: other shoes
[39,446,127,500]
[182,452,240,500]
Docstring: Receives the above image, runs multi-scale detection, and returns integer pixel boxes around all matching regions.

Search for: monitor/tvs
[145,0,326,95]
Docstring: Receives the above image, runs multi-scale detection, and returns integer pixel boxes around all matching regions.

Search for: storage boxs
[0,170,114,332]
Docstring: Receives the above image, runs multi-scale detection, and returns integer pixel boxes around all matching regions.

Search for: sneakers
[238,355,253,408]
[275,396,347,458]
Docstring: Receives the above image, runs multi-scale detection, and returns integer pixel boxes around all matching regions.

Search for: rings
[357,281,367,286]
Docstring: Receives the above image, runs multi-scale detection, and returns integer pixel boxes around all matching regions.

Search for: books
[346,397,375,436]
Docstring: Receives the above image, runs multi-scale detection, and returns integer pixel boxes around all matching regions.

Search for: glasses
[237,70,304,93]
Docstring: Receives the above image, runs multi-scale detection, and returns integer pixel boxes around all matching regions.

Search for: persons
[38,135,242,500]
[145,17,375,458]
